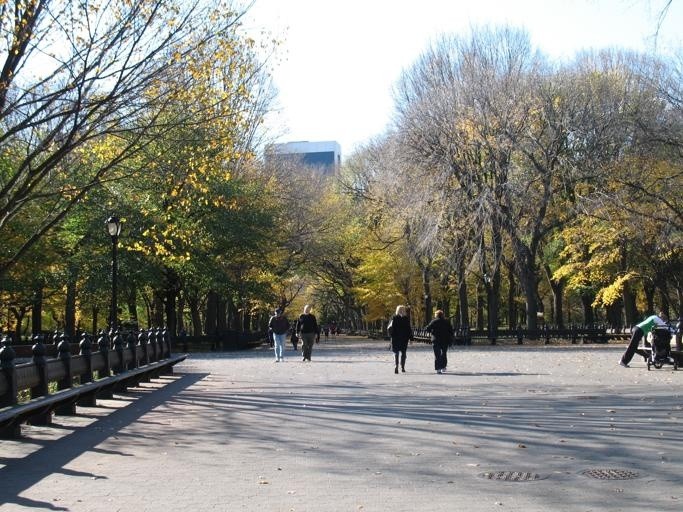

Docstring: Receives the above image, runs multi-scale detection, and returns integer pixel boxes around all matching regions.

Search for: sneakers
[303,356,311,361]
[276,357,284,361]
[395,367,405,373]
[437,368,445,373]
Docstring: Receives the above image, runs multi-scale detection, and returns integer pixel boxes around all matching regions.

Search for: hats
[275,308,282,314]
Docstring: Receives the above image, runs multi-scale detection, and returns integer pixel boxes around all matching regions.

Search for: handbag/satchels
[387,320,393,337]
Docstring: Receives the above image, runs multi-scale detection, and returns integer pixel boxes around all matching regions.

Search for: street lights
[104,217,123,350]
[484,272,493,338]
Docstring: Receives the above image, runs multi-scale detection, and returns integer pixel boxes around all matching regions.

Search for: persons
[619,311,667,368]
[267,306,342,362]
[387,305,414,373]
[426,310,453,374]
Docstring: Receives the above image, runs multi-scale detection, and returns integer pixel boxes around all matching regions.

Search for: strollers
[647,324,677,371]
